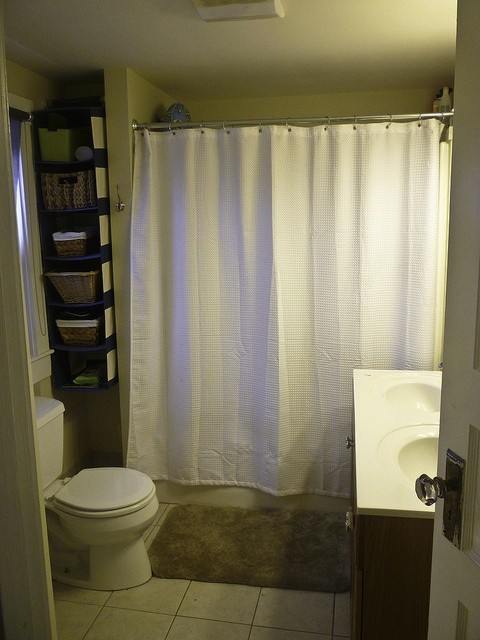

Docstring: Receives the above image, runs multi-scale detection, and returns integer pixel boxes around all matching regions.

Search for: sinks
[379,423,440,490]
[383,380,441,418]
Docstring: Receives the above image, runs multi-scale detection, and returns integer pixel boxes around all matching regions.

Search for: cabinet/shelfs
[31,107,118,393]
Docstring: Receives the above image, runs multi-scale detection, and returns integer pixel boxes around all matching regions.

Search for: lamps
[193,0,285,21]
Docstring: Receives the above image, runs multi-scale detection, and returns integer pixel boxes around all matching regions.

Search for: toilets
[34,395,159,592]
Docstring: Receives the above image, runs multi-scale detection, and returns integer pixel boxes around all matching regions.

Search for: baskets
[43,271,101,305]
[55,310,103,345]
[52,225,95,256]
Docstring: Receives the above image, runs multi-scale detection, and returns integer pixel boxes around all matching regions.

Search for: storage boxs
[38,127,77,163]
[41,170,96,210]
